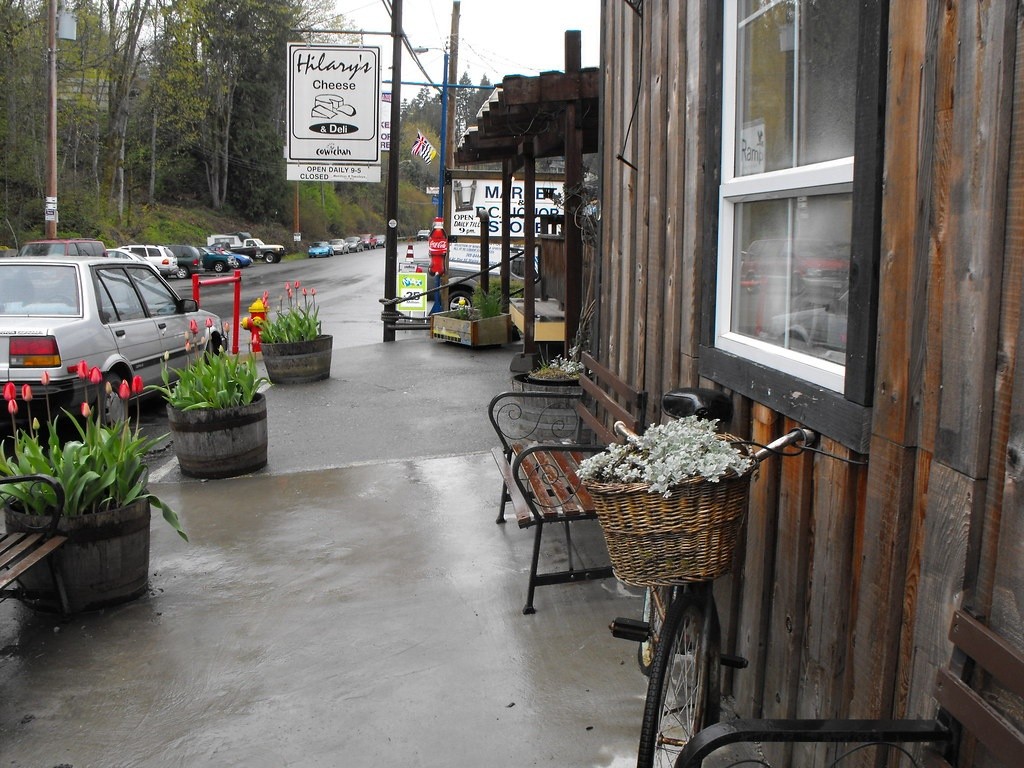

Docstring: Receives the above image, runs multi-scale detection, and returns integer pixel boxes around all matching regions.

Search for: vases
[167,391,267,480]
[4,484,152,617]
[261,333,333,384]
[510,373,588,444]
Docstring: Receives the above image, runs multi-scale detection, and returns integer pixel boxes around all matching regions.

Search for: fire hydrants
[240,298,270,353]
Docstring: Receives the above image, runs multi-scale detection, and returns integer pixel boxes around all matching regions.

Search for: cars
[374,235,386,248]
[0,256,228,440]
[105,249,160,275]
[308,241,335,258]
[345,237,364,252]
[195,246,253,273]
[329,238,350,255]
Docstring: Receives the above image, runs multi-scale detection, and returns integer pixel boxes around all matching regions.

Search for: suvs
[165,243,205,279]
[118,244,179,279]
[417,230,431,241]
[18,238,109,256]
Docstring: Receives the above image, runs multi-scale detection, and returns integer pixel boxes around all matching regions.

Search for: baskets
[579,431,756,587]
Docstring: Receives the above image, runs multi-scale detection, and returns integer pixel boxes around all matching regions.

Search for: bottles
[427,216,448,276]
[458,299,467,320]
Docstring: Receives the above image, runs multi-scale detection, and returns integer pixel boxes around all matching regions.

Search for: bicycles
[581,386,816,768]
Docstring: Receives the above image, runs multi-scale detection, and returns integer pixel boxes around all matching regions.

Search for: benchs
[676,609,1024,768]
[0,470,71,645]
[488,352,648,616]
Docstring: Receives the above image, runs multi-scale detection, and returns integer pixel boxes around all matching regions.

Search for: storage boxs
[431,308,511,349]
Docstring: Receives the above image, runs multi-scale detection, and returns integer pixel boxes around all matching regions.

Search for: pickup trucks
[359,234,377,250]
[232,238,285,264]
[211,242,256,259]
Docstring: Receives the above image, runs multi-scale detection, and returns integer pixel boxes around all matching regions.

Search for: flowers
[142,314,275,414]
[530,340,592,381]
[0,359,193,542]
[458,299,467,320]
[576,414,760,499]
[255,280,320,344]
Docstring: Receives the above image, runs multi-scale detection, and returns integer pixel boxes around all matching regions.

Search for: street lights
[412,45,457,309]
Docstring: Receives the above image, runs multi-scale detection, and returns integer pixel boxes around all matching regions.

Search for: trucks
[207,232,251,247]
[441,166,567,311]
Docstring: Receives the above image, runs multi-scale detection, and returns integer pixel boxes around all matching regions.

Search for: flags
[411,130,436,166]
